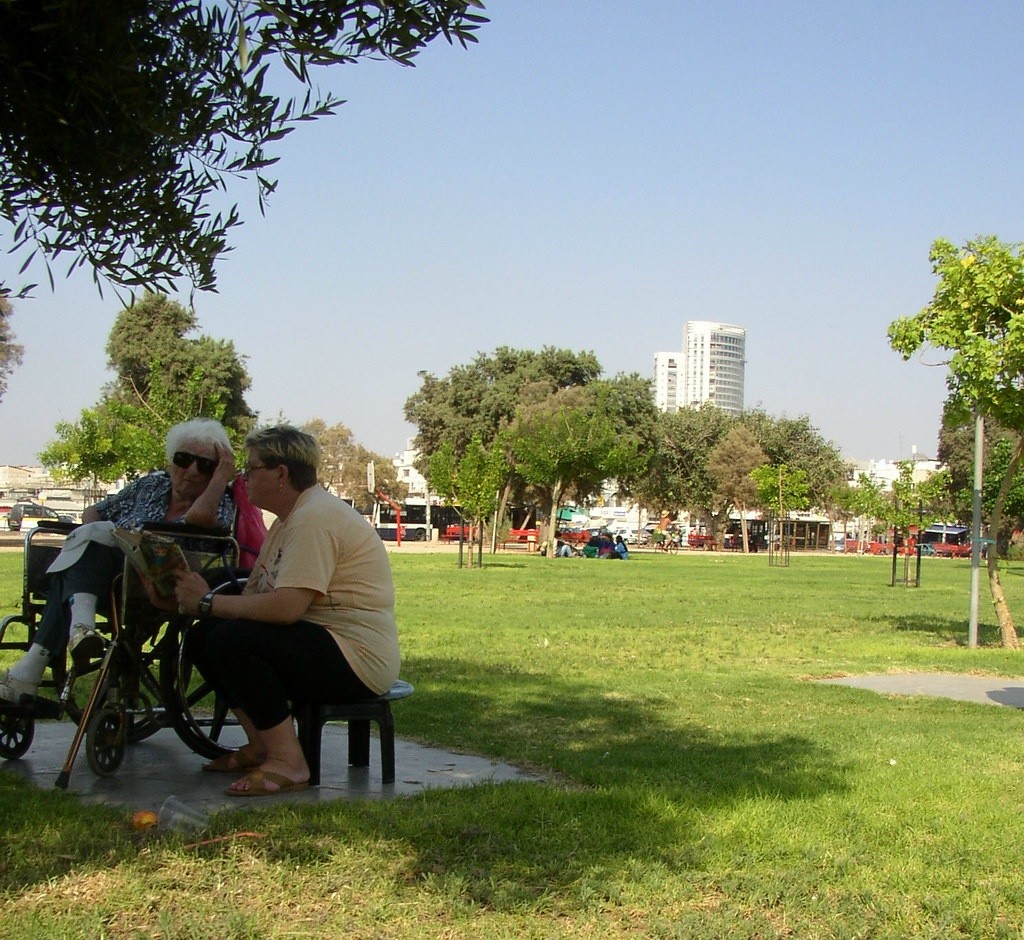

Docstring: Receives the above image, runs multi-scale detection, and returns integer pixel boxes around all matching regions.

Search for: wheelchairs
[1,516,258,778]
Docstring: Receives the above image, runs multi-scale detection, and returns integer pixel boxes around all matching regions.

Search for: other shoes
[70,632,105,669]
[0,671,37,705]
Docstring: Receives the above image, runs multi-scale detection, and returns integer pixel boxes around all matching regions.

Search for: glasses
[172,451,220,476]
[244,461,271,475]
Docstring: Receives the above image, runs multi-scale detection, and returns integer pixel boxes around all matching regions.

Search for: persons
[171,424,400,798]
[733,530,743,551]
[0,418,235,706]
[539,529,630,559]
[657,509,675,551]
[677,528,683,547]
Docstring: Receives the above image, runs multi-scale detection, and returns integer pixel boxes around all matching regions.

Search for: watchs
[198,592,214,618]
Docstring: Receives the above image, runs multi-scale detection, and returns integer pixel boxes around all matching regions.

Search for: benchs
[496,530,539,552]
[560,533,591,548]
[441,526,478,545]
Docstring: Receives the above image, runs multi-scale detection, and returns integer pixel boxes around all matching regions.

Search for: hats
[45,521,119,574]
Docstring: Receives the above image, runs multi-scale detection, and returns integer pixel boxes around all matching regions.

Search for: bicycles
[652,526,680,556]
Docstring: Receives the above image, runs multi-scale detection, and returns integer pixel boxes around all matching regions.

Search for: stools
[296,678,415,786]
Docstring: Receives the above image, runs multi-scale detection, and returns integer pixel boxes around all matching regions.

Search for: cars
[834,538,846,553]
[612,528,654,545]
[8,503,78,531]
[583,528,609,539]
[914,543,936,556]
[560,527,578,535]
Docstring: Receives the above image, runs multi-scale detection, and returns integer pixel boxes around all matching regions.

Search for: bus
[372,497,538,543]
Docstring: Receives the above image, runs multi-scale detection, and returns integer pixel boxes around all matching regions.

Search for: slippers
[224,767,309,796]
[202,753,261,773]
